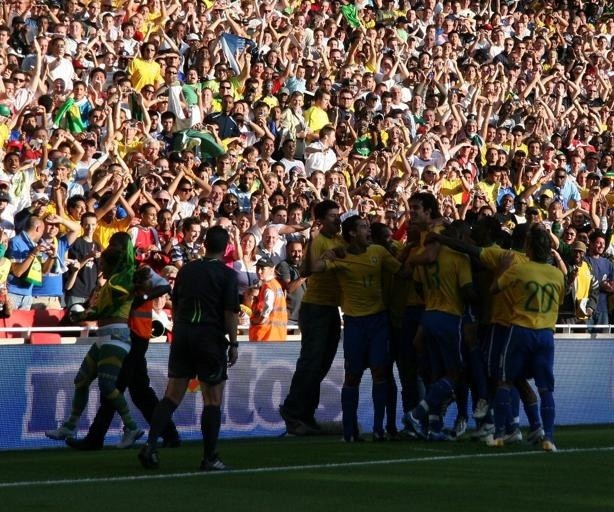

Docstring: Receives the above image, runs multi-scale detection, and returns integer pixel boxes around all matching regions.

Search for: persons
[279,191,567,453]
[44,231,182,452]
[131,223,243,470]
[0,1,614,342]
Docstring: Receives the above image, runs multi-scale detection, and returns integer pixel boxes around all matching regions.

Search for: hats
[253,257,274,267]
[572,241,587,253]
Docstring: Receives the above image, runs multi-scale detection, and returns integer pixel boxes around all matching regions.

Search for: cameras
[335,185,340,193]
[155,167,162,174]
[122,88,133,94]
[247,168,253,171]
[369,184,378,190]
[225,225,235,232]
[475,190,484,196]
[85,132,93,139]
[128,122,138,129]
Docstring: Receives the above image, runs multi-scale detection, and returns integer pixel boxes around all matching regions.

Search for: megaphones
[150,320,165,337]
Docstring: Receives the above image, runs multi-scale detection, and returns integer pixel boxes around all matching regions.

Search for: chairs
[0,305,175,346]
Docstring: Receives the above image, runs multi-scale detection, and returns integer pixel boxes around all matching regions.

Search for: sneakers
[200,453,234,471]
[157,434,182,449]
[343,398,523,448]
[279,405,324,436]
[543,439,557,452]
[45,426,77,441]
[116,426,145,450]
[65,437,103,452]
[525,426,546,445]
[138,442,161,470]
